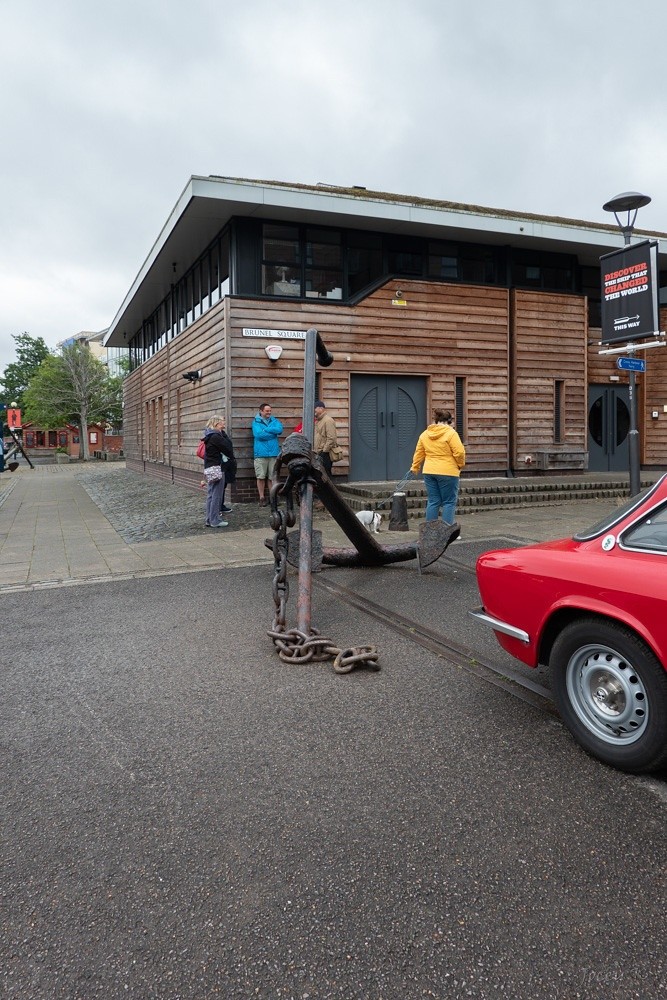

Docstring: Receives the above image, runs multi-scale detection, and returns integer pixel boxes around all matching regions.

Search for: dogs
[355,510,383,534]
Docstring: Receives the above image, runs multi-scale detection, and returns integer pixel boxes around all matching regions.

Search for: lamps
[396,285,403,298]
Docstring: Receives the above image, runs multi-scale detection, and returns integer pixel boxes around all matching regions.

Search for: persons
[315,401,338,481]
[252,404,283,506]
[410,408,465,540]
[204,415,233,527]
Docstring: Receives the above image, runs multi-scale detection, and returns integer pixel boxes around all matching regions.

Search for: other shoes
[205,520,229,528]
[260,498,267,507]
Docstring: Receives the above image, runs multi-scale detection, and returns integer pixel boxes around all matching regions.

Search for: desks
[266,282,301,296]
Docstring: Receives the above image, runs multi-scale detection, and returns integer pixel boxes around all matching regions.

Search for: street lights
[601,189,652,498]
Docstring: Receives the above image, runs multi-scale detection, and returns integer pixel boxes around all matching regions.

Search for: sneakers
[220,505,232,512]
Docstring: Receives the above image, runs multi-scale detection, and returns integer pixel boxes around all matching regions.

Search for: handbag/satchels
[203,466,222,483]
[329,443,343,462]
[197,441,205,459]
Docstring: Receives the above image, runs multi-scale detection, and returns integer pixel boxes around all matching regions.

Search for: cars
[465,469,667,778]
